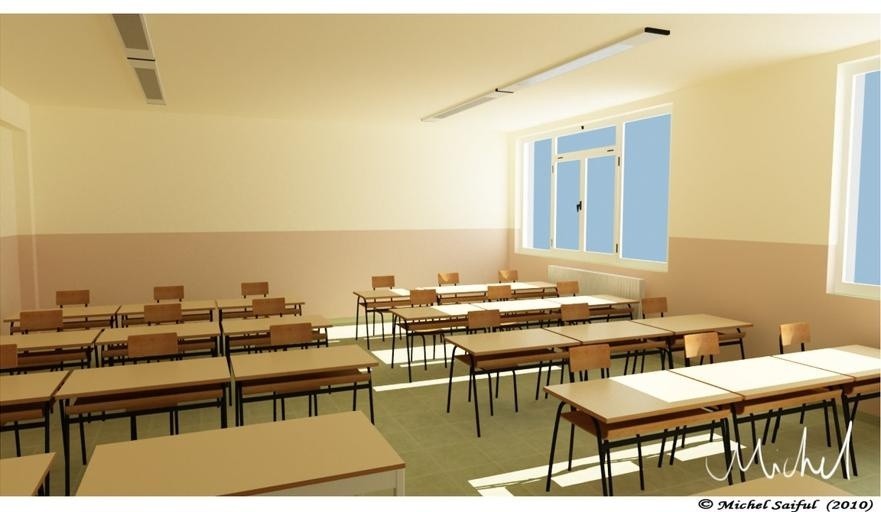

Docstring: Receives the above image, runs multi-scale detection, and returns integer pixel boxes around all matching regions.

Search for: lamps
[497,25,672,100]
[112,14,160,62]
[125,59,168,108]
[418,85,516,127]
